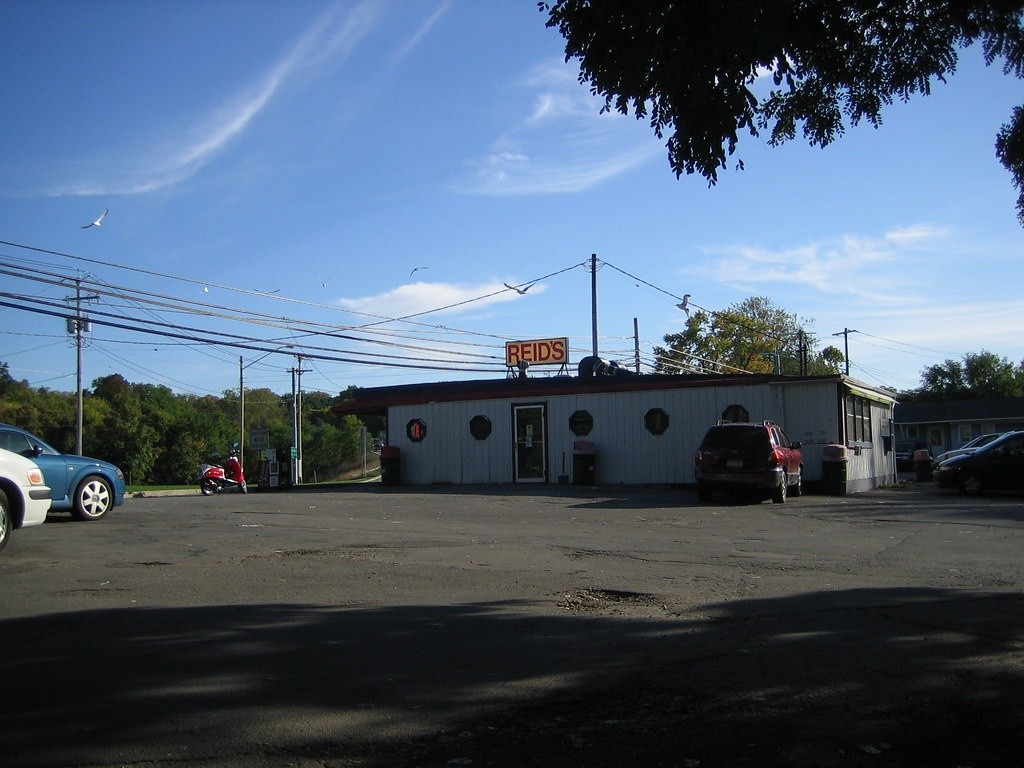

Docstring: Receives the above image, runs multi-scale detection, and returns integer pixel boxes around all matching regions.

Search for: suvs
[694,419,805,504]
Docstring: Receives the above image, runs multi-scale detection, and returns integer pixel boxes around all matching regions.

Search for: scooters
[195,442,248,496]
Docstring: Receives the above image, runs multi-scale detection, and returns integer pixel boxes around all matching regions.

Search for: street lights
[240,343,296,471]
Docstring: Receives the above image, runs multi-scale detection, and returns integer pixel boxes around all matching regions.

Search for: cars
[932,430,1024,499]
[0,446,54,553]
[895,440,917,467]
[933,432,1006,469]
[0,423,127,522]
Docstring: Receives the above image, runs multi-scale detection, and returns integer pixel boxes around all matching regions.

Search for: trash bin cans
[573,453,596,487]
[913,459,933,483]
[821,457,848,497]
[380,454,401,484]
